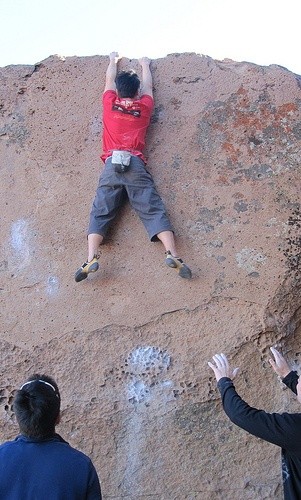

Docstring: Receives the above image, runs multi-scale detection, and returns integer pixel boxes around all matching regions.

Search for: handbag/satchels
[111,151,131,173]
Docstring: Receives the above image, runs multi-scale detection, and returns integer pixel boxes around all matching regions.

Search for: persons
[206,346,301,500]
[0,374,102,500]
[74,52,193,281]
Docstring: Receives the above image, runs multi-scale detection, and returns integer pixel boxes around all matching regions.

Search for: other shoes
[165,250,191,280]
[74,256,99,282]
